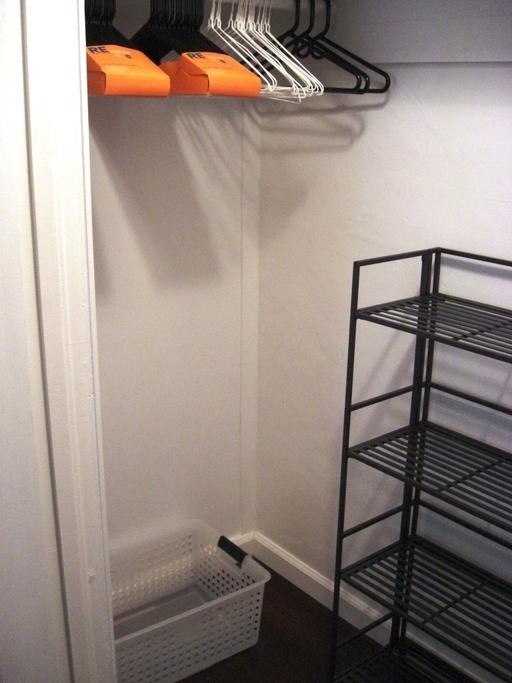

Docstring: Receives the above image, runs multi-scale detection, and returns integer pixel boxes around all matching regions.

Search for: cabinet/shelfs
[324,246,512,683]
